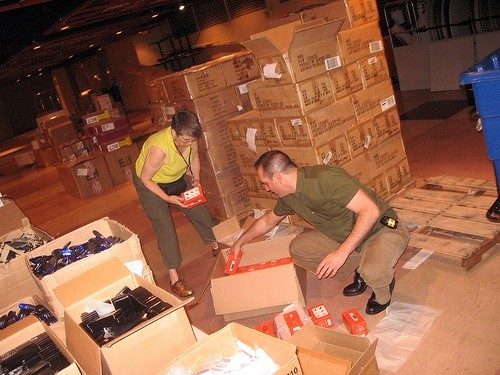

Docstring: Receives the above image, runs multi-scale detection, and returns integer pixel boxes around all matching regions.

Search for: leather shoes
[343,266,368,297]
[365,277,395,315]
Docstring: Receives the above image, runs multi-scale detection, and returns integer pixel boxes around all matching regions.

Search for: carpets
[401,96,468,122]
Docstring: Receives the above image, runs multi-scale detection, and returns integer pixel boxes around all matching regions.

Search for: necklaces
[174,142,195,184]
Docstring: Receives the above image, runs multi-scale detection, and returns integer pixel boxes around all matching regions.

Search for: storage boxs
[0,0,412,375]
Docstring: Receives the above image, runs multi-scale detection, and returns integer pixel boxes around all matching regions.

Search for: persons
[133,110,221,298]
[225,150,410,315]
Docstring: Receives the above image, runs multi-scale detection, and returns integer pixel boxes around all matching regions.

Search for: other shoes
[211,244,219,257]
[169,279,194,297]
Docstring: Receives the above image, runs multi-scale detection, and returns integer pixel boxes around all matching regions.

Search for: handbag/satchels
[157,175,187,196]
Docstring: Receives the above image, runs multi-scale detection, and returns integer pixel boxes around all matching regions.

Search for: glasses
[177,134,197,144]
